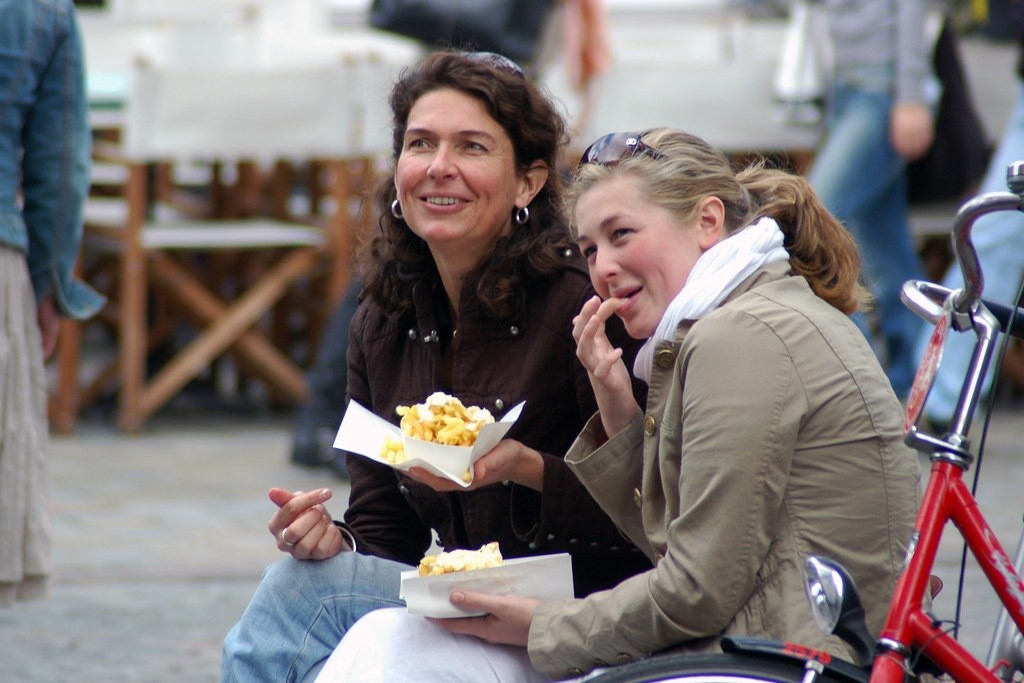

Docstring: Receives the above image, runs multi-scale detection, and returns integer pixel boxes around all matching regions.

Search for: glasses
[460,50,524,81]
[576,130,695,178]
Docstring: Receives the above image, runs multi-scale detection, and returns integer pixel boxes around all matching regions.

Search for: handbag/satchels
[901,16,987,206]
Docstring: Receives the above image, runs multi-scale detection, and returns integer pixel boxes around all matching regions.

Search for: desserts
[397,391,495,481]
[418,540,502,577]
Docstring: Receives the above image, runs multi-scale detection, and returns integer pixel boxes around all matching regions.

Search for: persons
[219,48,648,683]
[802,0,1024,441]
[0,0,108,604]
[314,127,932,683]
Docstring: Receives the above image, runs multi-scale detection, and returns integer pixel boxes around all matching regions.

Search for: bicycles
[582,158,1024,683]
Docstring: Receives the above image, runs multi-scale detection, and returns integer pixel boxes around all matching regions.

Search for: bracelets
[336,526,356,552]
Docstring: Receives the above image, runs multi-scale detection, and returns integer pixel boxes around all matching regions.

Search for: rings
[282,528,294,546]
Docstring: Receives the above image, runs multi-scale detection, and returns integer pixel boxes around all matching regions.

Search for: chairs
[46,52,375,424]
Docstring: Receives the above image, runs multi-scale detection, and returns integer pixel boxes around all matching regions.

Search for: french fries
[380,436,404,465]
